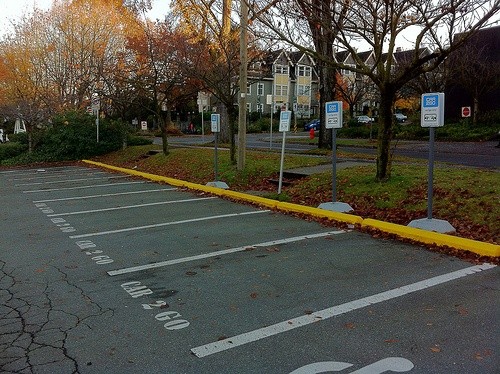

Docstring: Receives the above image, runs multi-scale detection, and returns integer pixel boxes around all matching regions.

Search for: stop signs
[87,106,92,113]
[141,121,147,131]
[461,106,471,117]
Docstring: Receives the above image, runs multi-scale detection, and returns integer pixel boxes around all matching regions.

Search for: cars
[393,113,407,123]
[354,116,375,124]
[304,119,320,131]
